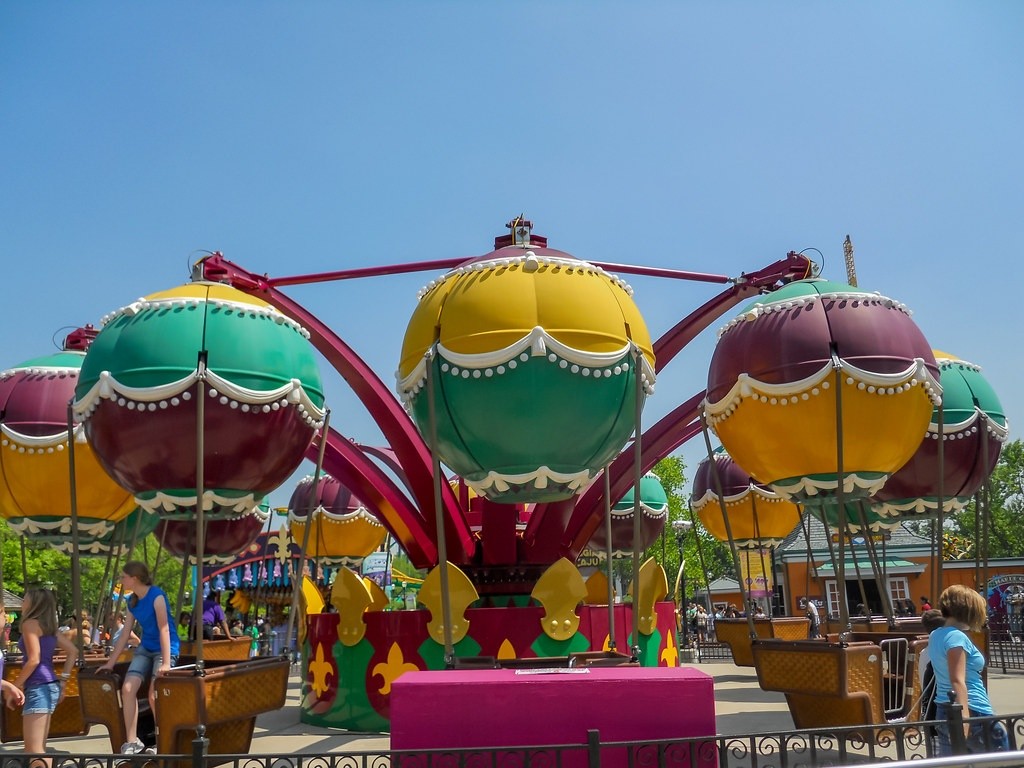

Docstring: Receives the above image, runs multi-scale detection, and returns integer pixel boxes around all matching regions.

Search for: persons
[176,591,300,659]
[0,593,26,705]
[928,584,1009,758]
[95,560,180,766]
[800,597,822,639]
[894,596,931,617]
[58,609,111,646]
[678,602,767,643]
[918,609,945,756]
[6,588,78,768]
[108,611,141,649]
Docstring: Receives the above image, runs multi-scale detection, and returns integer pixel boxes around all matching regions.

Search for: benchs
[0,637,287,762]
[714,608,990,744]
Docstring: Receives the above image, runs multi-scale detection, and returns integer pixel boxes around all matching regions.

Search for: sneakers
[114,736,145,767]
[145,744,158,759]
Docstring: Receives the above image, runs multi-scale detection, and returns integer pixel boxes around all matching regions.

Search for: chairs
[453,656,501,670]
[568,650,639,668]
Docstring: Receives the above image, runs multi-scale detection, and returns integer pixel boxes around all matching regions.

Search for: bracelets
[61,672,71,678]
[60,679,67,682]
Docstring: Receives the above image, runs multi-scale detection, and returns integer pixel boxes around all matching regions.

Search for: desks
[389,667,717,768]
